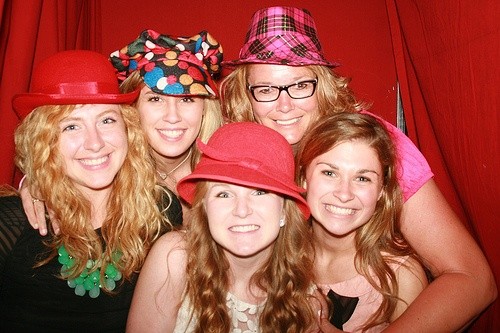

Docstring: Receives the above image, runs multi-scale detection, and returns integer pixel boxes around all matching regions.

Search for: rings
[33,199,39,203]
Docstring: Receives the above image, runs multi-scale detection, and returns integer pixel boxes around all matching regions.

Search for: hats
[221,7,340,69]
[176,122,310,220]
[108,30,224,100]
[11,50,140,123]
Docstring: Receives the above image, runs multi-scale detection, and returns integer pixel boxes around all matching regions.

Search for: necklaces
[57,240,127,298]
[155,148,193,180]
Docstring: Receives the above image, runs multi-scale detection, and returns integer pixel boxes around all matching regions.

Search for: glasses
[246,76,318,102]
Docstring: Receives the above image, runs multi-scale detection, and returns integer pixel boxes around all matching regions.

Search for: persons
[296,112,429,333]
[18,30,224,236]
[218,6,497,333]
[0,50,184,333]
[126,122,330,333]
